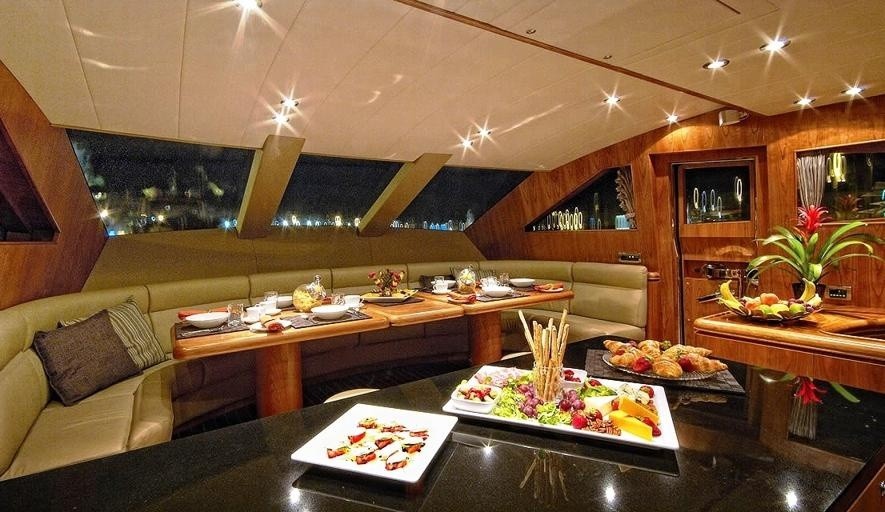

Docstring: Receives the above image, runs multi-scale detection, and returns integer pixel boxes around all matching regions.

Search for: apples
[757,304,805,312]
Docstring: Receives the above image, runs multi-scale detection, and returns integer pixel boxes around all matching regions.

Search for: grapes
[789,297,819,311]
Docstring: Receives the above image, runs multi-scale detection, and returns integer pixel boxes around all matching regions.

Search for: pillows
[32,310,143,407]
[58,294,171,371]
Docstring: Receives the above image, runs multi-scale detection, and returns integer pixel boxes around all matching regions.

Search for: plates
[290,403,460,484]
[602,335,718,381]
[440,363,681,453]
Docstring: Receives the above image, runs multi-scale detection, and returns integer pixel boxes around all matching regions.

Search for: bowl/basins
[184,278,536,330]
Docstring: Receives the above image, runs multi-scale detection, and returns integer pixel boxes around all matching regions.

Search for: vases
[794,282,826,301]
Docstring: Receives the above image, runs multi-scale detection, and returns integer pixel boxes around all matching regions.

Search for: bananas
[720,280,743,308]
[798,277,821,306]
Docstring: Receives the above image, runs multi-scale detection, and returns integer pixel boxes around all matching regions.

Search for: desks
[693,301,885,447]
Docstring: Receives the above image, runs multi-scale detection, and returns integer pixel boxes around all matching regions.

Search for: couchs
[0,261,647,482]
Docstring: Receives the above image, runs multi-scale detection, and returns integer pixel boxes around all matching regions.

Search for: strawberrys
[457,370,662,443]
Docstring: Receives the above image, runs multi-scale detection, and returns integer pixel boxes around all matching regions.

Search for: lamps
[718,109,752,126]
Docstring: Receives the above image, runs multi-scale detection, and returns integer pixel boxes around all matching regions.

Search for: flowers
[745,203,885,289]
[368,269,405,296]
[835,195,861,220]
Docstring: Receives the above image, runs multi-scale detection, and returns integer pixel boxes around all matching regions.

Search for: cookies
[518,307,569,401]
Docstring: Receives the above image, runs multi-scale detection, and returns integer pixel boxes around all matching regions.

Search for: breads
[604,339,729,379]
[327,417,428,470]
